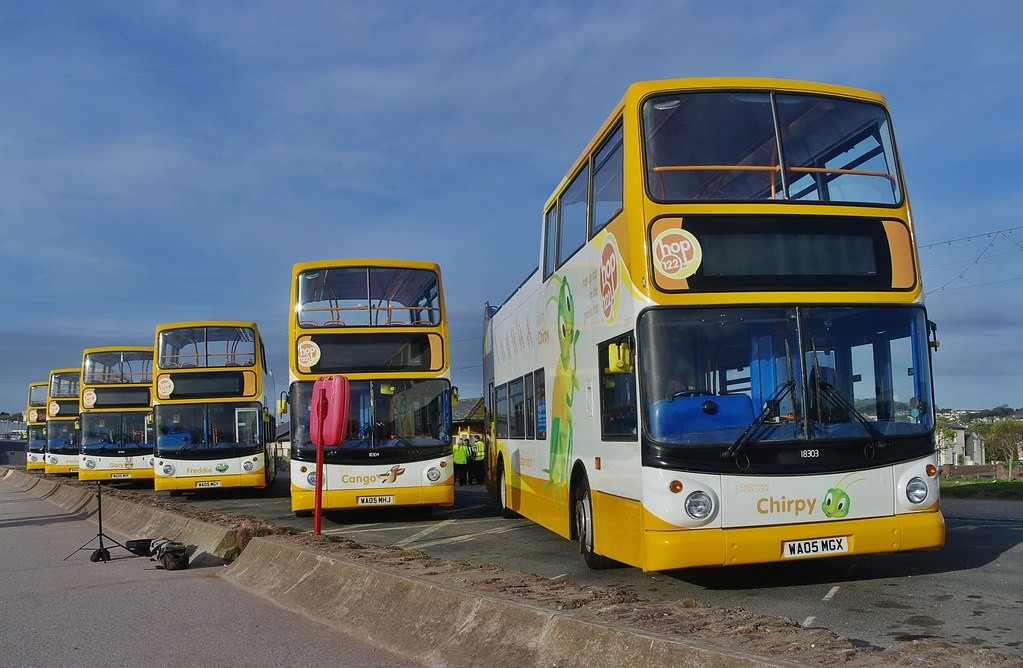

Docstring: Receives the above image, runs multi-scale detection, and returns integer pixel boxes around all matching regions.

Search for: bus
[22,381,80,470]
[43,368,114,477]
[481,79,946,574]
[276,259,459,518]
[146,319,277,497]
[74,345,154,484]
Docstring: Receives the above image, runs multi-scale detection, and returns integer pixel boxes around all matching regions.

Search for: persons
[453,435,486,486]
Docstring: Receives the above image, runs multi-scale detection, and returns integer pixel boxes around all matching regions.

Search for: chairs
[299,320,432,326]
[89,378,152,383]
[162,360,254,369]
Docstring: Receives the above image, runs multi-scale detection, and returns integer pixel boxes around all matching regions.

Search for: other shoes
[460,484,464,486]
[469,482,473,485]
